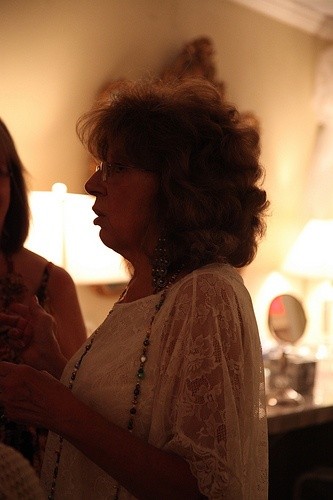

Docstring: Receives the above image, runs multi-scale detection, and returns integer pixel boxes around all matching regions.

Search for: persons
[0,76,270,500]
[0,114,87,471]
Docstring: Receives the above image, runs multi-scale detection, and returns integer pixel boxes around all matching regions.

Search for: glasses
[0,167,14,186]
[96,161,149,184]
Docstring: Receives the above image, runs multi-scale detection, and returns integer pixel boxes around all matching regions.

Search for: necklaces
[47,263,184,500]
